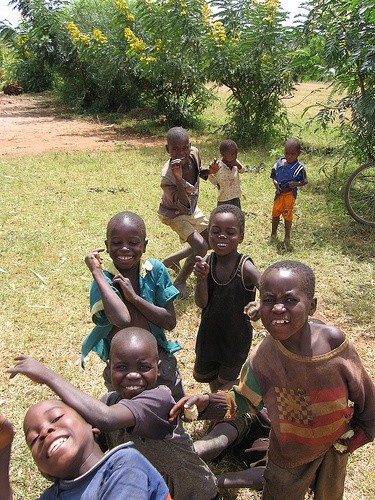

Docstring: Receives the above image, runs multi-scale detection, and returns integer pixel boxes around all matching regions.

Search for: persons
[0,399,173,500]
[208,140,246,210]
[193,203,263,394]
[5,327,225,500]
[270,138,309,241]
[156,126,222,301]
[168,260,375,500]
[85,211,185,403]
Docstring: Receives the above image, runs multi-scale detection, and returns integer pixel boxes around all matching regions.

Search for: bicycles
[344,159,375,229]
